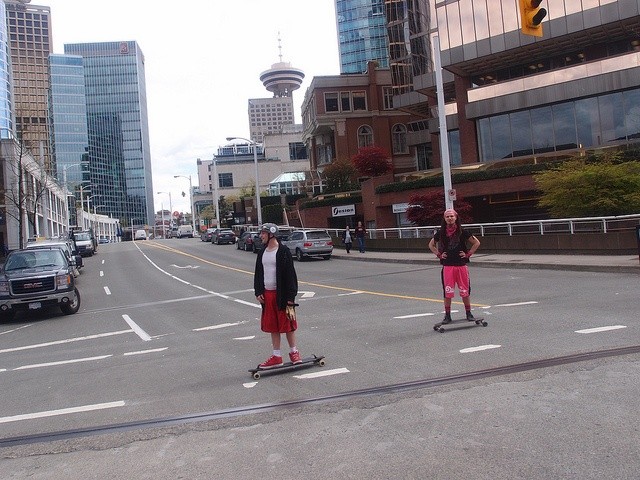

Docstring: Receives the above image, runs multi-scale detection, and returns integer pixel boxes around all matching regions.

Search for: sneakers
[467,313,475,321]
[442,316,451,324]
[289,351,303,365]
[257,354,283,370]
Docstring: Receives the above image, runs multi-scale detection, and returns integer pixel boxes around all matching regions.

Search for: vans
[178,225,192,238]
[135,230,146,239]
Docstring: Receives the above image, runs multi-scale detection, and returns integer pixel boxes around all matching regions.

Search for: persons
[253,222,303,369]
[341,225,353,253]
[429,209,480,325]
[354,221,367,253]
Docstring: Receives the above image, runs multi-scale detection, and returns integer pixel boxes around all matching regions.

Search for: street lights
[80,184,97,231]
[174,175,196,237]
[94,205,105,219]
[131,218,135,241]
[87,194,102,227]
[158,192,173,226]
[227,137,262,229]
[64,160,89,233]
[196,159,220,230]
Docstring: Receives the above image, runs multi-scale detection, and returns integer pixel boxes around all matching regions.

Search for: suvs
[74,233,94,256]
[167,228,177,239]
[26,243,75,280]
[281,228,333,261]
[0,248,80,322]
[253,227,291,253]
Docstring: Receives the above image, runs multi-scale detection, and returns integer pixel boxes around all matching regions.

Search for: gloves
[285,304,300,321]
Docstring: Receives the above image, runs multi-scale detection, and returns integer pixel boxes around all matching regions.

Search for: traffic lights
[519,0,546,37]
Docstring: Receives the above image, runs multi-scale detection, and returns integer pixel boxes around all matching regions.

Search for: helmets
[258,222,279,238]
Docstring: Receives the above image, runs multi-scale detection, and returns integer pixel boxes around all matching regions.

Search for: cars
[236,232,258,251]
[201,229,214,241]
[212,228,235,245]
[99,239,109,244]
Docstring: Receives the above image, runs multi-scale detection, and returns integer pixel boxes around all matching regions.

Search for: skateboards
[433,317,488,333]
[248,355,326,380]
